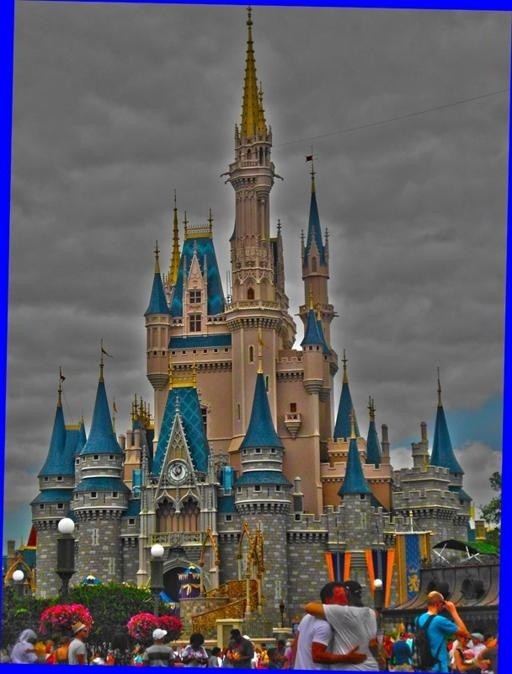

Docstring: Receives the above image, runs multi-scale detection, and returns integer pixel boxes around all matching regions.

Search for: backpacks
[412,615,445,672]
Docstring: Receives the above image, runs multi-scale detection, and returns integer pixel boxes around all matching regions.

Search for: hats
[230,630,240,639]
[152,628,167,640]
[470,632,484,642]
[71,622,86,634]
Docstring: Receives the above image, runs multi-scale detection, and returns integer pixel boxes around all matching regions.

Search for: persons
[10,581,499,674]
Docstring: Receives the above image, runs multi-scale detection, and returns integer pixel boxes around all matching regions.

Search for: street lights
[53,516,78,606]
[146,543,164,616]
[370,579,384,630]
[10,570,28,601]
